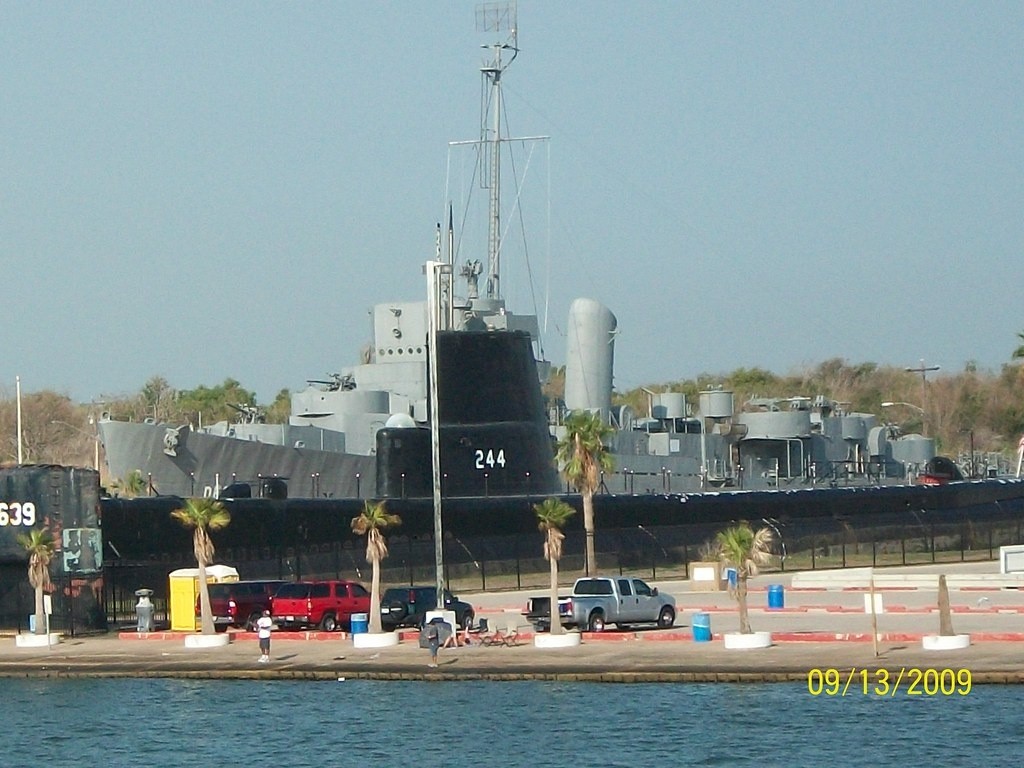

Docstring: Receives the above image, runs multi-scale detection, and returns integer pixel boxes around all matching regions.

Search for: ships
[0,465,1024,628]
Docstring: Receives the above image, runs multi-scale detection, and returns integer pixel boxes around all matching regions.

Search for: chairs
[477,619,499,646]
[500,621,518,648]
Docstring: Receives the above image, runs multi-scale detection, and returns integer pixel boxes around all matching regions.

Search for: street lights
[882,402,930,437]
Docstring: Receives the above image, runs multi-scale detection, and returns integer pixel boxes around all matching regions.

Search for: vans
[194,579,288,633]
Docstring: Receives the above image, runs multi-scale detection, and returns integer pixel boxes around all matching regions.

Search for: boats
[79,2,939,497]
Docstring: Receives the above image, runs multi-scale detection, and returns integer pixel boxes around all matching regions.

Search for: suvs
[380,586,476,632]
[270,581,371,632]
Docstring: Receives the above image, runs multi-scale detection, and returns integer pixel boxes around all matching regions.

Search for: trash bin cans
[351,613,368,640]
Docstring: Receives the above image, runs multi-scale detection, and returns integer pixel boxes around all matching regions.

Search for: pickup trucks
[521,577,677,632]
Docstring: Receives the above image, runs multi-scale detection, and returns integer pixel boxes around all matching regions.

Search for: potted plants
[690,536,728,592]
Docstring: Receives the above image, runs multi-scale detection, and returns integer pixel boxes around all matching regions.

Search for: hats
[428,620,435,626]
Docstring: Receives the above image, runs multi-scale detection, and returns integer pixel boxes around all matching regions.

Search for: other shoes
[428,663,438,667]
[257,657,270,663]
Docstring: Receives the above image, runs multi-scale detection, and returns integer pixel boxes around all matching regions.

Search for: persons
[426,620,439,667]
[254,610,272,663]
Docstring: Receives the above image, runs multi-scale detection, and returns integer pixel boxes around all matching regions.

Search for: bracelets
[429,636,430,638]
